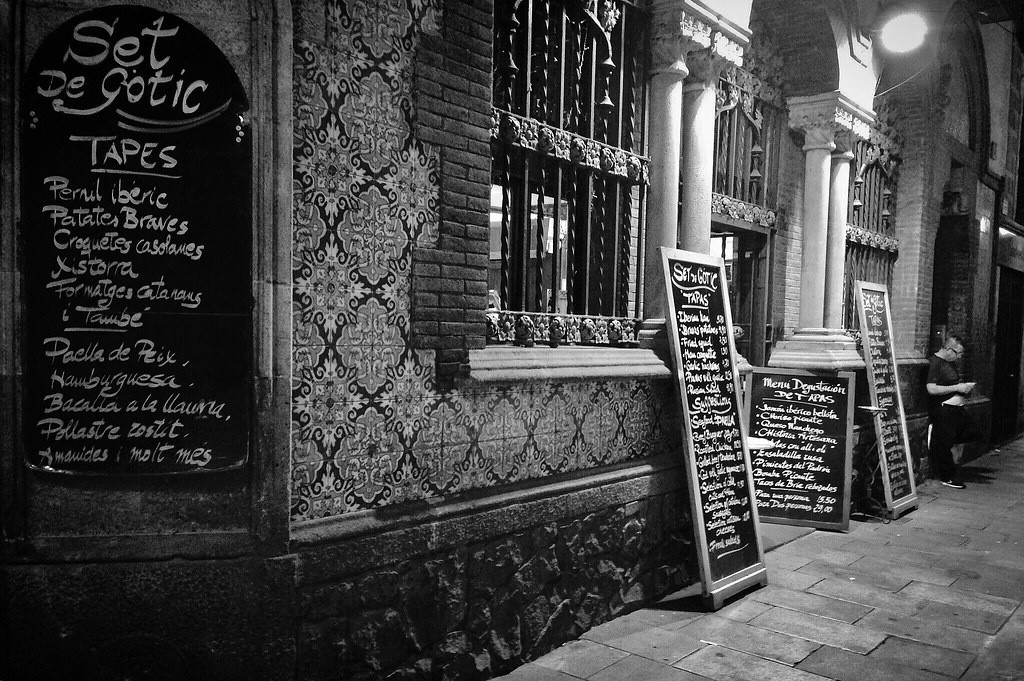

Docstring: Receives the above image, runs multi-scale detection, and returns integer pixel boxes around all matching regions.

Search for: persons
[926,335,974,488]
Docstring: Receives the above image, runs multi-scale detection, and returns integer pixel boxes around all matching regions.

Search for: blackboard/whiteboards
[659,245,765,597]
[11,2,257,483]
[743,367,856,531]
[855,279,916,511]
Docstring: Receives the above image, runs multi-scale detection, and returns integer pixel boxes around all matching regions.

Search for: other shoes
[928,424,935,452]
[941,479,964,488]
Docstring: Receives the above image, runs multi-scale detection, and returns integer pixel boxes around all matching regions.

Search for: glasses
[947,348,963,359]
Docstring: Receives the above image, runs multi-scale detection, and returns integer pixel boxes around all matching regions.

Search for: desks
[849,407,891,524]
